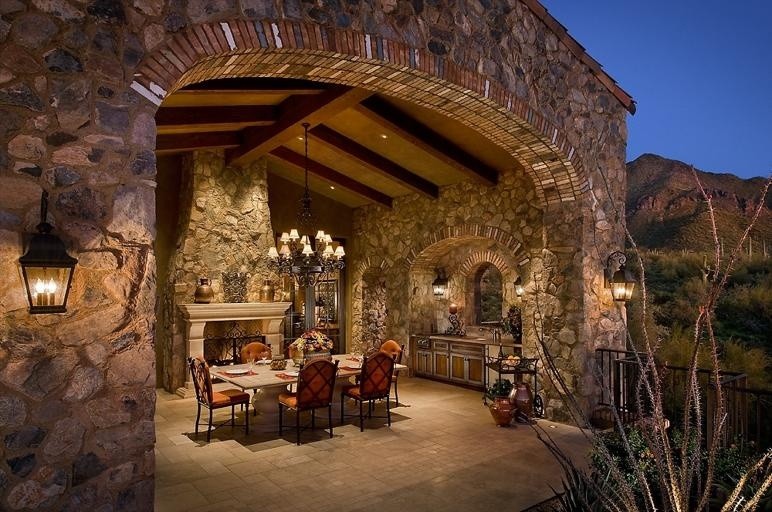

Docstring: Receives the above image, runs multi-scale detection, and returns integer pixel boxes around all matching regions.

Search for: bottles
[260,280,274,303]
[195,279,214,304]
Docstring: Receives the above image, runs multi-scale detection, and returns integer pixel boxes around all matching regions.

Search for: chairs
[185,326,406,447]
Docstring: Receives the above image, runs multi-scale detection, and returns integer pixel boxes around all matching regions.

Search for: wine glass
[260,352,269,368]
[246,357,256,376]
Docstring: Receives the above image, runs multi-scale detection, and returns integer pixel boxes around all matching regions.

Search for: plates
[285,372,299,377]
[226,369,248,374]
[257,360,271,365]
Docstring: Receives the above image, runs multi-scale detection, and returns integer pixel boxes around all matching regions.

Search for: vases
[489,381,533,428]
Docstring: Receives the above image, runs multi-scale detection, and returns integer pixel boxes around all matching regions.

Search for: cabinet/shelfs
[415,350,433,380]
[433,341,450,385]
[448,343,486,392]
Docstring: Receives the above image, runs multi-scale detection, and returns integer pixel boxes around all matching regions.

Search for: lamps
[263,118,347,288]
[604,250,637,303]
[513,276,522,298]
[432,272,448,296]
[16,190,79,315]
